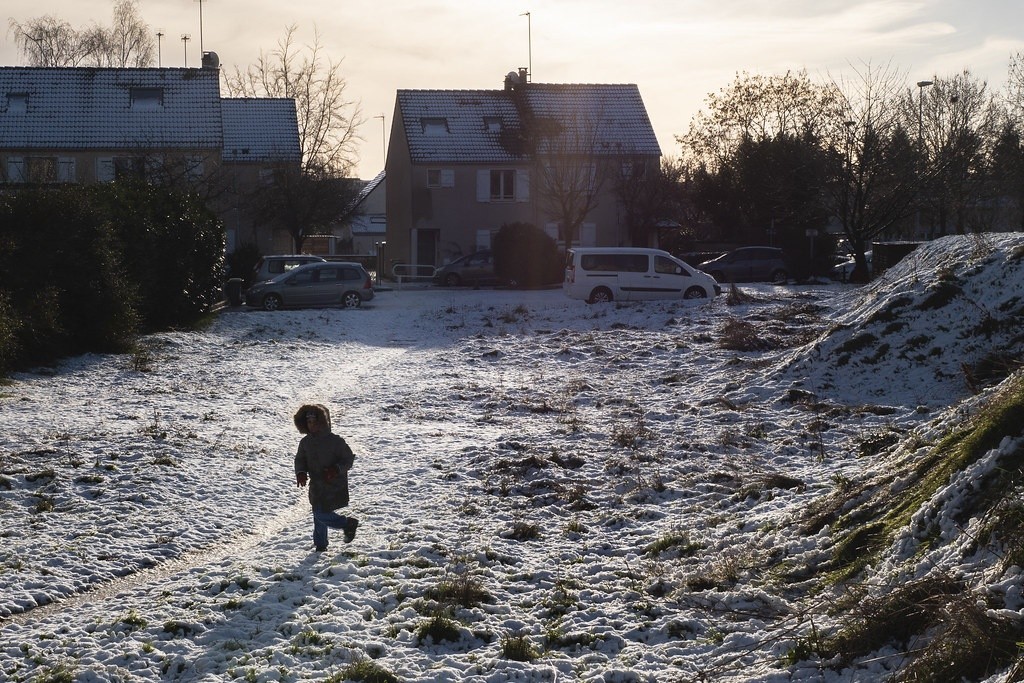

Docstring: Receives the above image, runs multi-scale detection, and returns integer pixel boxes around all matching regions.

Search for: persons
[293,404,358,551]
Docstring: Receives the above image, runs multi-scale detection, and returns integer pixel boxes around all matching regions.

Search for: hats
[304,412,318,419]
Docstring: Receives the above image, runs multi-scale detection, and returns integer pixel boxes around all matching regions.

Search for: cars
[831,248,872,283]
[697,246,797,283]
[434,249,502,288]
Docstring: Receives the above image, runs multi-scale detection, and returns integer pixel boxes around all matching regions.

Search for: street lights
[917,78,934,177]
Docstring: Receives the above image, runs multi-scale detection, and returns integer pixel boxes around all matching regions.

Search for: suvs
[246,262,374,311]
[253,255,327,282]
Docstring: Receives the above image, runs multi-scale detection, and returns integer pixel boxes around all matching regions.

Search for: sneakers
[343,517,358,543]
[315,545,326,553]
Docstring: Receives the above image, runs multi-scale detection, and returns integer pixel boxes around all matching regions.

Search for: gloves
[321,464,343,481]
[297,472,308,487]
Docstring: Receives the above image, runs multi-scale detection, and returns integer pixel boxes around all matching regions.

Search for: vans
[562,247,722,304]
